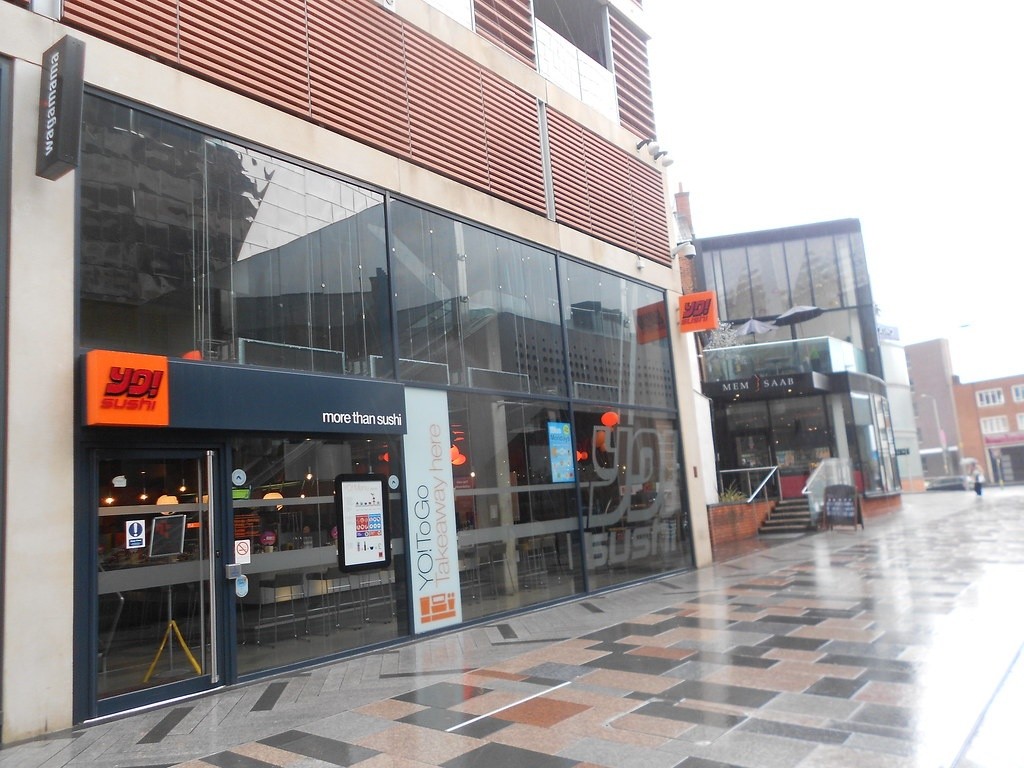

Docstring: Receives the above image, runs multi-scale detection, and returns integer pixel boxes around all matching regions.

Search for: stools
[129,535,562,645]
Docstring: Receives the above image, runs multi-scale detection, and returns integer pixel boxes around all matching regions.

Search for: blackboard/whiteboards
[824,485,862,527]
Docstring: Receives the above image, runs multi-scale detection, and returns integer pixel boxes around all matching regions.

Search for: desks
[765,355,790,376]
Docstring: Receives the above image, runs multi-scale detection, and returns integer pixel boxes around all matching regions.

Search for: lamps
[637,138,660,155]
[156,495,178,515]
[653,151,674,168]
[265,492,284,511]
[451,445,466,465]
[576,411,619,462]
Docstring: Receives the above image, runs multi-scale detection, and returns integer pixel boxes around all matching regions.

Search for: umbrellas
[959,457,979,465]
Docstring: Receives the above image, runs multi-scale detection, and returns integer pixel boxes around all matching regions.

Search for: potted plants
[809,348,822,372]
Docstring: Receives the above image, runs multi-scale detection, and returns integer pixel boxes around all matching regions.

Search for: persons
[970,464,984,496]
[303,507,329,610]
